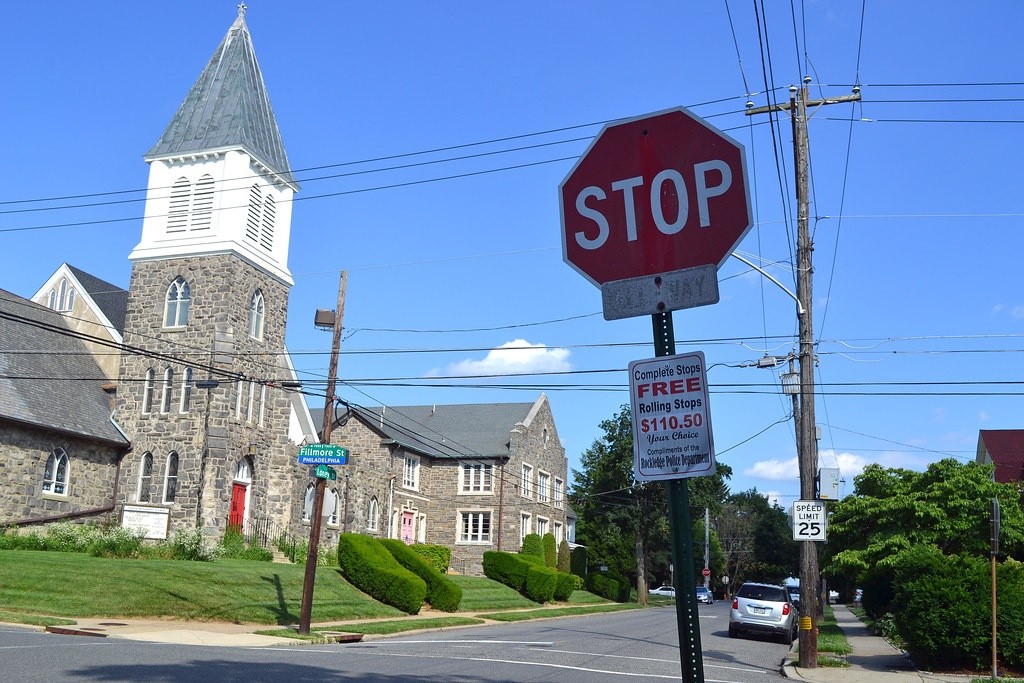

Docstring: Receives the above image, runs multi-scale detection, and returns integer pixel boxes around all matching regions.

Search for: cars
[648,586,675,598]
[696,586,713,604]
[784,584,800,603]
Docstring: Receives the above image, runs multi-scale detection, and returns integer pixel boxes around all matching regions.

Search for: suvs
[729,582,798,645]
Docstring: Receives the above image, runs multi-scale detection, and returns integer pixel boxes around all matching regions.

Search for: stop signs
[702,568,711,577]
[558,106,754,291]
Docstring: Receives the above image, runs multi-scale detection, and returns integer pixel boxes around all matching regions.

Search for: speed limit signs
[794,500,828,542]
